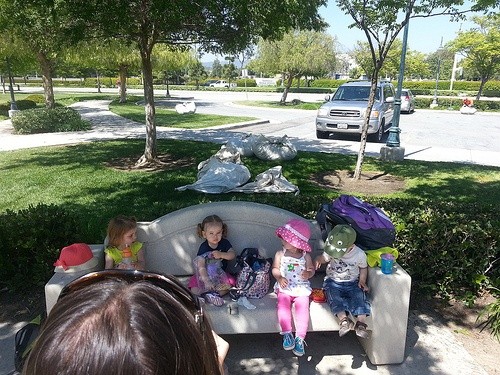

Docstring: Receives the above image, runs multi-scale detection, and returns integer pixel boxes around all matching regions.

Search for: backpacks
[14,311,47,372]
[315,194,396,250]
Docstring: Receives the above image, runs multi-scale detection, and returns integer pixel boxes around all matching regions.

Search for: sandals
[338,317,355,337]
[355,320,368,338]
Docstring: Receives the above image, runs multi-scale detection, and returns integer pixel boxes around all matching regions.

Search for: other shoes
[197,296,205,305]
[292,337,304,356]
[203,291,224,306]
[283,332,295,350]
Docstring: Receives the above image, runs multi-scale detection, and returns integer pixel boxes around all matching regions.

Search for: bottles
[206,253,217,276]
[122,244,132,271]
[380,253,396,274]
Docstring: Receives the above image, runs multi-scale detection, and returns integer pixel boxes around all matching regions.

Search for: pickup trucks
[210,80,238,88]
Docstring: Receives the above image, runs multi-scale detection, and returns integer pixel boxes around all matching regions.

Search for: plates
[309,287,327,303]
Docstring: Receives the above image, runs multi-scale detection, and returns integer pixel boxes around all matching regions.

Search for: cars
[204,80,218,86]
[394,89,415,114]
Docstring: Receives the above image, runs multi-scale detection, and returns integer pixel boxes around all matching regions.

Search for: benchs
[45,201,411,364]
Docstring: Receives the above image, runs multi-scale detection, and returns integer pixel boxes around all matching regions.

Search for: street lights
[429,36,444,109]
[450,22,463,90]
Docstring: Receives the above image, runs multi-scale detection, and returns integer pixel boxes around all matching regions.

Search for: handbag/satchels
[235,250,272,298]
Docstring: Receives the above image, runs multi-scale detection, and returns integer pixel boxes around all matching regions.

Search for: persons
[312,224,372,338]
[186,215,237,306]
[272,219,315,357]
[103,215,146,271]
[12,269,224,375]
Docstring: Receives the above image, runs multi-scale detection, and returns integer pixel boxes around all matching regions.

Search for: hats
[275,218,312,252]
[53,243,99,273]
[323,223,356,258]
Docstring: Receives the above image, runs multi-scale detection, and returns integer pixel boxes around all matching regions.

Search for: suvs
[315,78,396,142]
